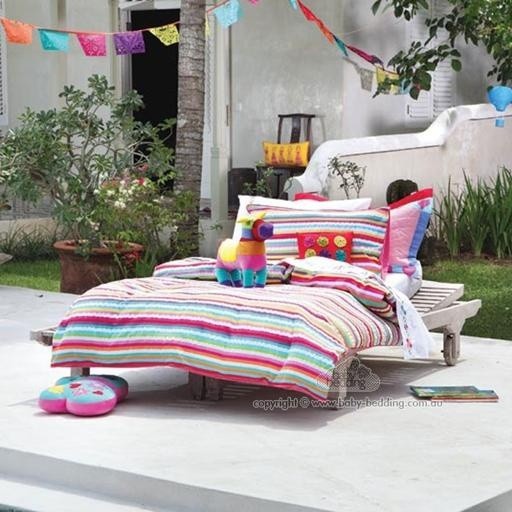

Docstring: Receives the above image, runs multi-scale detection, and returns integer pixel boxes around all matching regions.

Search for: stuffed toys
[214,211,277,290]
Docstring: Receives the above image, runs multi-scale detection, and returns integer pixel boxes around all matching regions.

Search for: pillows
[263,141,311,169]
[228,187,436,300]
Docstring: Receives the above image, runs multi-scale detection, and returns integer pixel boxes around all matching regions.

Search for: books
[410,383,480,397]
[431,390,499,403]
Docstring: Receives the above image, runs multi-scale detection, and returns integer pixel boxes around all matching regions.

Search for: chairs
[254,113,317,199]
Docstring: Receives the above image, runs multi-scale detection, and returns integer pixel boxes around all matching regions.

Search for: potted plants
[0,72,179,295]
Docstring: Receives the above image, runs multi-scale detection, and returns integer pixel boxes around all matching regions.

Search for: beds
[27,254,484,414]
